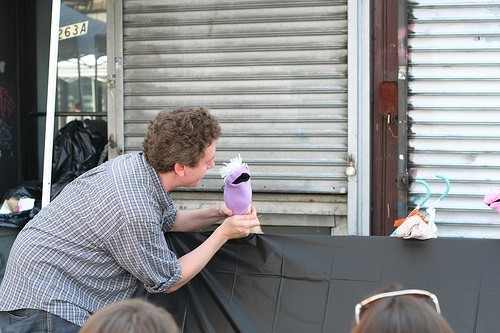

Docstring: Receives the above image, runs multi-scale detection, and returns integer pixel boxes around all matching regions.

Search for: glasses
[355,289,441,325]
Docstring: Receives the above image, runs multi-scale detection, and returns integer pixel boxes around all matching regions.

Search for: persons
[351,285,455,333]
[78,297,180,333]
[0,106,260,333]
